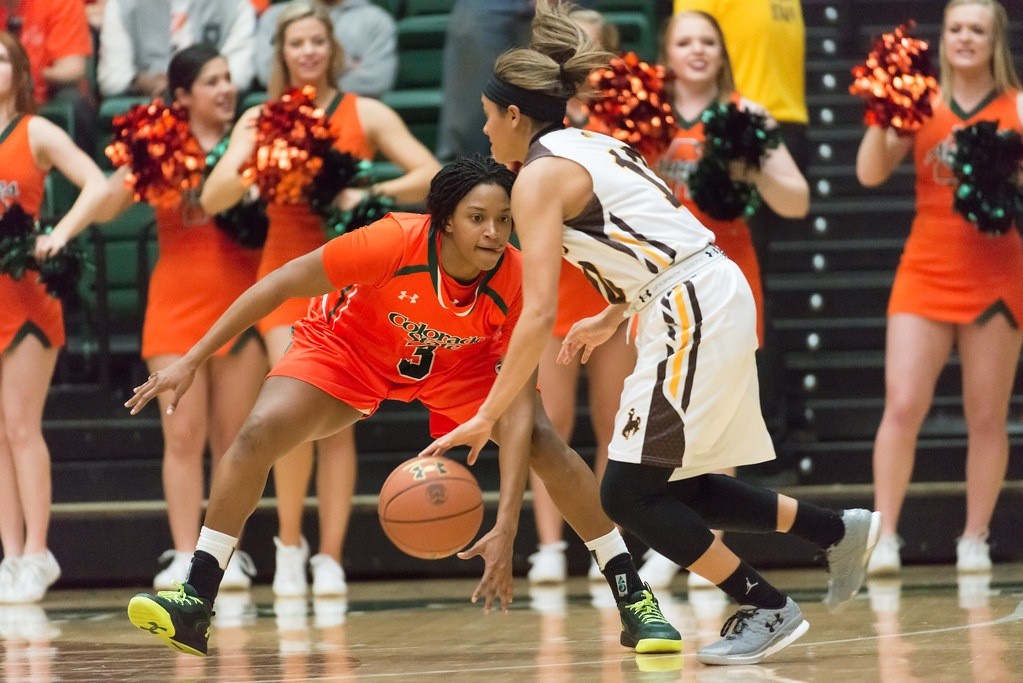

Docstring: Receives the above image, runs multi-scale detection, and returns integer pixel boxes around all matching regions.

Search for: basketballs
[377,455,485,560]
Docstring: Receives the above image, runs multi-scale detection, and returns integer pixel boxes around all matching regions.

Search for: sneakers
[687,572,715,587]
[621,654,683,683]
[127,580,216,657]
[688,588,729,618]
[529,586,567,617]
[823,508,882,613]
[590,582,617,609]
[869,533,902,576]
[309,554,347,596]
[957,541,992,572]
[637,548,680,589]
[696,596,810,665]
[0,605,61,642]
[867,578,900,611]
[590,557,606,580]
[618,581,684,653]
[527,541,569,584]
[153,549,195,591]
[0,547,61,604]
[312,598,346,627]
[218,549,256,590]
[214,593,246,628]
[273,536,309,596]
[957,573,992,609]
[274,598,307,629]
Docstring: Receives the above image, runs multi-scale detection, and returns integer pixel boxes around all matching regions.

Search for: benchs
[0,0,1023,487]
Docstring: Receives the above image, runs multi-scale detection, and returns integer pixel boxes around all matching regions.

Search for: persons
[422,0,881,667]
[0,0,812,658]
[855,0,1023,576]
